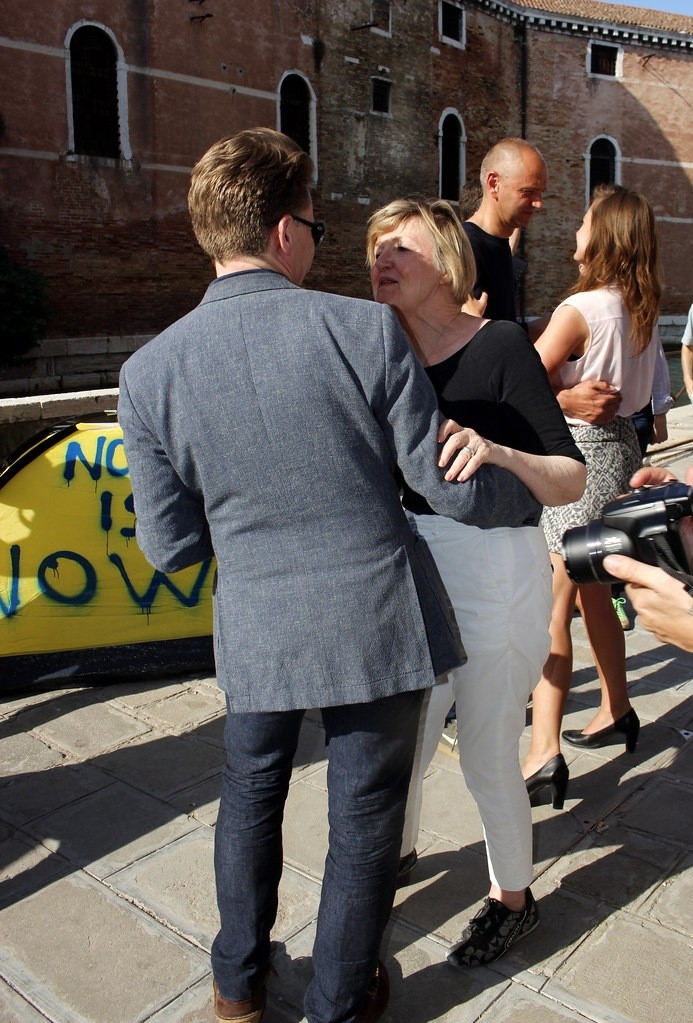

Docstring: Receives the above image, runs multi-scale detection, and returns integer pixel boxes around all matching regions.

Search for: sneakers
[612,596,630,630]
[447,886,539,968]
[397,849,419,878]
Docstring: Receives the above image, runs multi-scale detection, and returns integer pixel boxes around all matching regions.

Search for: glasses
[270,214,327,248]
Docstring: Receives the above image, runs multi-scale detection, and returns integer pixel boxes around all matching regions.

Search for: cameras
[562,481,693,585]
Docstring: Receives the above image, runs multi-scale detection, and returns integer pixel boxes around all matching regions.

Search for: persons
[366,199,588,970]
[461,186,658,810]
[604,467,693,654]
[680,305,693,403]
[443,138,625,745]
[612,332,675,629]
[118,128,544,1023]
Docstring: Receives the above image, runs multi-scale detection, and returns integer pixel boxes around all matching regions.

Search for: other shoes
[212,976,265,1023]
[357,958,390,1022]
[442,719,459,746]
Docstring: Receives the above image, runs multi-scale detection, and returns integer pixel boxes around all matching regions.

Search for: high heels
[562,708,639,754]
[524,753,569,810]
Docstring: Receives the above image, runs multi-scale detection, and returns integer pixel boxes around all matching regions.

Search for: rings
[464,446,474,457]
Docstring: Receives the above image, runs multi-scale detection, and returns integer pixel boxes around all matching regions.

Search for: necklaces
[423,312,461,365]
